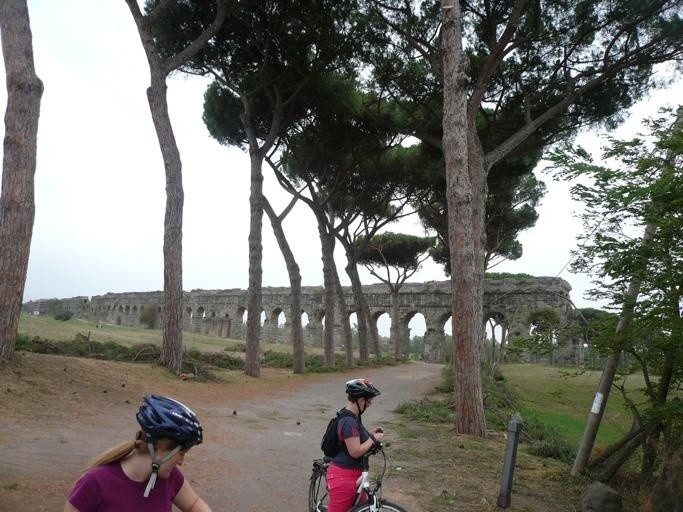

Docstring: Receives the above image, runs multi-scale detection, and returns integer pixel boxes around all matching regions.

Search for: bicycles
[307,440,408,512]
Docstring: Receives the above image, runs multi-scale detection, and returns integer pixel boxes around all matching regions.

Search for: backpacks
[321,412,361,459]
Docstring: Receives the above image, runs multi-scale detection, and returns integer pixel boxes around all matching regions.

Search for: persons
[326,379,384,512]
[64,394,212,512]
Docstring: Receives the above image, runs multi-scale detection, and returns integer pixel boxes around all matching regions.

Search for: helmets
[136,394,203,445]
[345,378,381,397]
